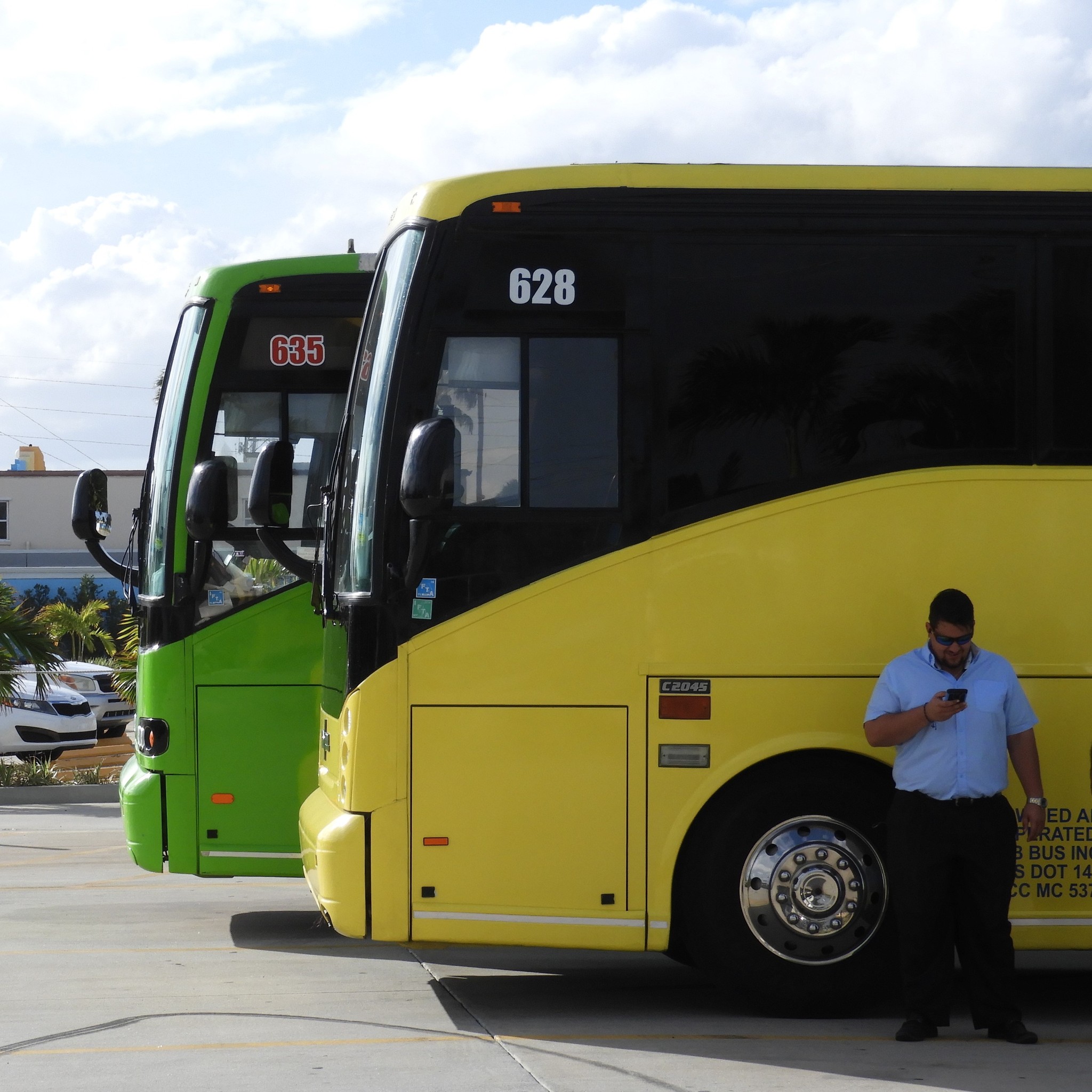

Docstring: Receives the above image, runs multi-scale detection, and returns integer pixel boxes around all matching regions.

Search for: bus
[246,162,1092,1020]
[70,237,523,878]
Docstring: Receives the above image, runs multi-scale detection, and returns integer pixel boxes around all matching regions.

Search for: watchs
[1027,796,1048,808]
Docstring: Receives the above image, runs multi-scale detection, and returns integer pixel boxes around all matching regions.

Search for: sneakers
[989,1021,1037,1044]
[894,1018,939,1041]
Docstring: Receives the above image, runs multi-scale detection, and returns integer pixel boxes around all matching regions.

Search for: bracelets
[924,703,936,730]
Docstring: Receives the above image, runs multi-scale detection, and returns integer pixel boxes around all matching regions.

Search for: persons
[862,588,1044,1045]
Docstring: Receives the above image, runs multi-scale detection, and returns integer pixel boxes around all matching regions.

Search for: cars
[0,674,98,766]
[0,632,136,739]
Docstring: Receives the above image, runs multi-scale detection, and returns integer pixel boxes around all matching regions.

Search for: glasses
[930,625,972,646]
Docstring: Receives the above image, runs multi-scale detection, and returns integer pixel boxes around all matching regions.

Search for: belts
[929,798,990,807]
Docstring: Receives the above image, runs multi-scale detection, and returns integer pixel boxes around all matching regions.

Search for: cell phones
[944,688,968,702]
